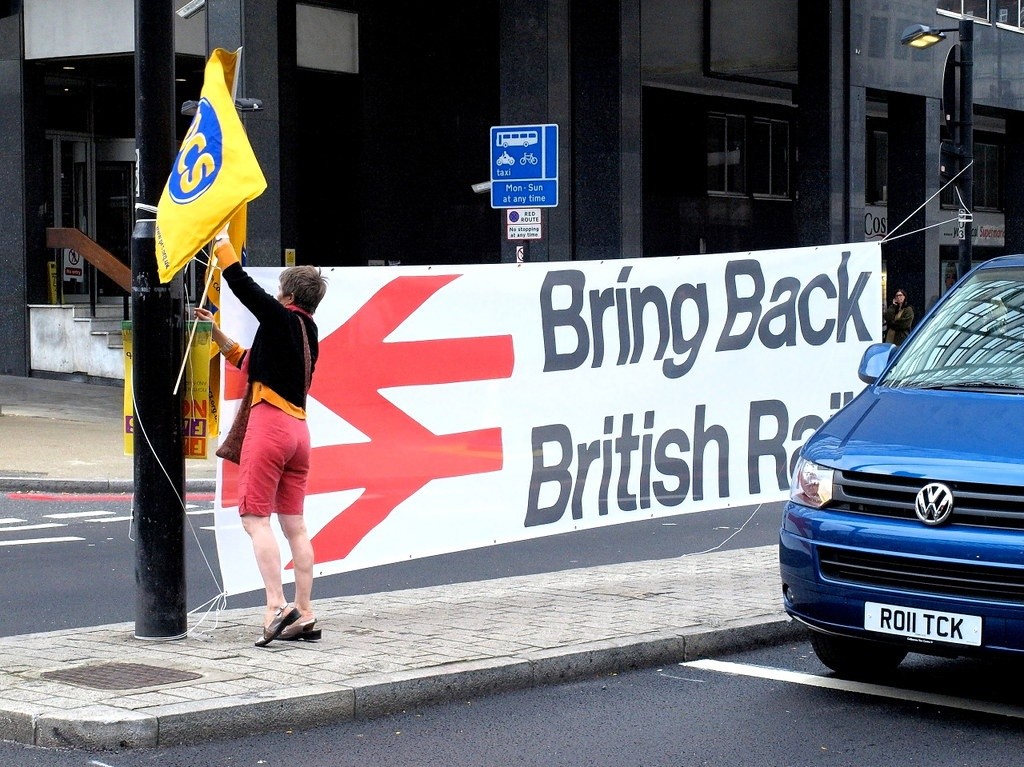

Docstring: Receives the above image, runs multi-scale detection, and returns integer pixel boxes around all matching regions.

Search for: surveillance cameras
[175,0,204,20]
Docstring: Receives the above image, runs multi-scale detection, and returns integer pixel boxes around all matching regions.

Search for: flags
[152,45,270,287]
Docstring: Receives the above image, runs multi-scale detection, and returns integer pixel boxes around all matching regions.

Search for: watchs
[215,234,229,243]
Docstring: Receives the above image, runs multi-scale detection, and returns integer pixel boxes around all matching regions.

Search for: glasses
[895,294,903,297]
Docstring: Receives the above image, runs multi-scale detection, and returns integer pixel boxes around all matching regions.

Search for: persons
[883,289,914,349]
[944,262,958,292]
[208,234,326,649]
[990,296,1007,335]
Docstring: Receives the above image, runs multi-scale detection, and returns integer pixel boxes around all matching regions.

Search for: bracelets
[219,339,236,354]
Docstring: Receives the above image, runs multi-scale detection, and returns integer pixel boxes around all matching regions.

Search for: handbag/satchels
[215,381,254,467]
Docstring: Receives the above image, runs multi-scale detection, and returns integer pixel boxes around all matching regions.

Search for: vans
[780,253,1024,699]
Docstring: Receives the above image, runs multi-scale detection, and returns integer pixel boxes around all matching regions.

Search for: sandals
[275,618,322,641]
[254,603,301,646]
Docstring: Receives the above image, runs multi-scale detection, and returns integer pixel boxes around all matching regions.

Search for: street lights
[903,16,974,288]
